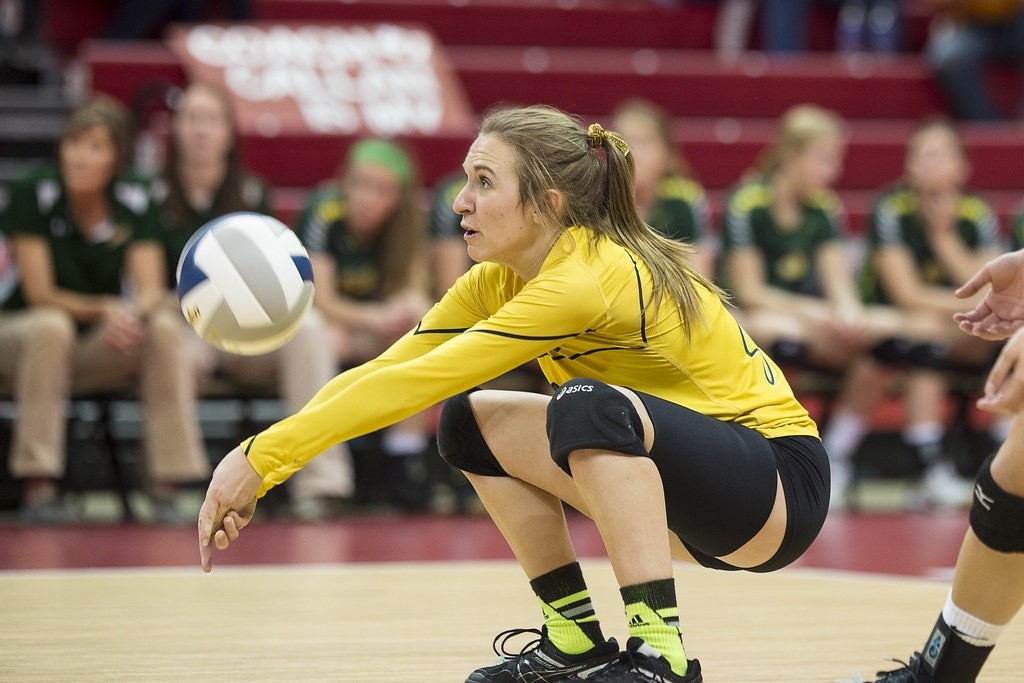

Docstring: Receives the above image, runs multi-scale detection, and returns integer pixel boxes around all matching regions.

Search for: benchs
[38,0,1024,432]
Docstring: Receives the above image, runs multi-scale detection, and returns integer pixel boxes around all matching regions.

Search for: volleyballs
[175,211,315,358]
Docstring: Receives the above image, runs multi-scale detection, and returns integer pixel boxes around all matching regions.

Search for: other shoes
[922,463,976,515]
[25,492,77,526]
[825,455,854,511]
[142,470,186,527]
[294,493,347,520]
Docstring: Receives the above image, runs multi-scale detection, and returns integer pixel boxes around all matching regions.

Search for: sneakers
[463,627,619,683]
[570,637,704,683]
[858,650,927,683]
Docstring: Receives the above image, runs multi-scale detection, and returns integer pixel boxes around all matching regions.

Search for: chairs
[0,382,408,523]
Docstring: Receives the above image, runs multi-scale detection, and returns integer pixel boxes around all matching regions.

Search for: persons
[0,91,212,524]
[0,0,1024,523]
[854,248,1024,683]
[192,105,831,683]
